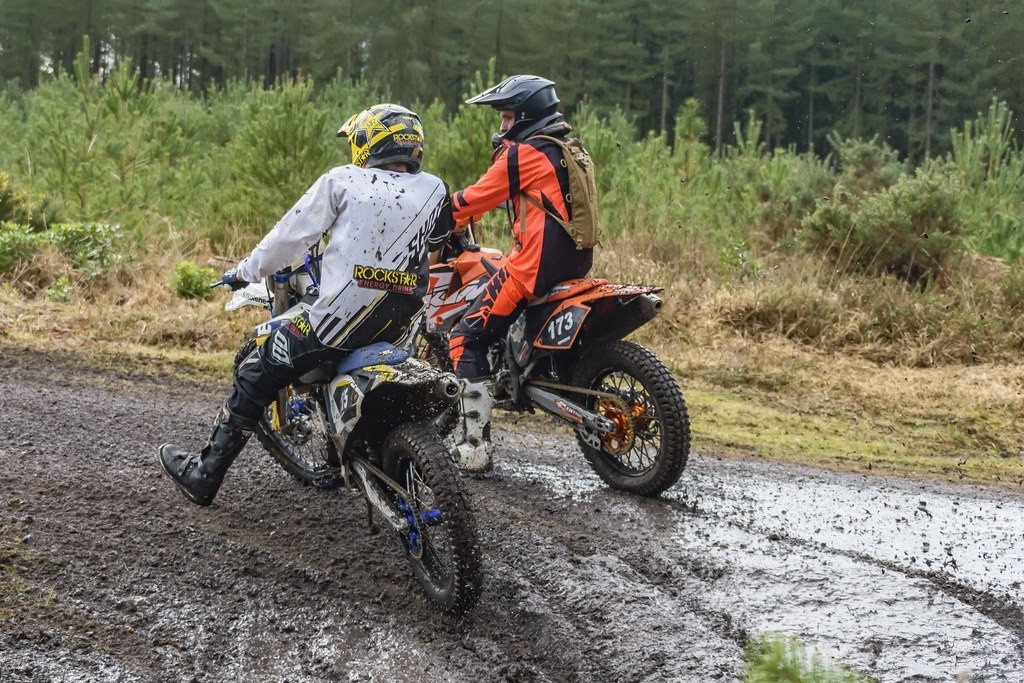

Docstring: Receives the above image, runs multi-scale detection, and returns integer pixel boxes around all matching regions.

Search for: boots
[158,399,258,513]
[443,376,494,471]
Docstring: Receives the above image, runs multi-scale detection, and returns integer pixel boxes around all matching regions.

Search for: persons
[159,102,455,508]
[447,74,596,473]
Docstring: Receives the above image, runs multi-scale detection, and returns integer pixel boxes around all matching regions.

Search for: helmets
[464,74,563,145]
[336,102,424,174]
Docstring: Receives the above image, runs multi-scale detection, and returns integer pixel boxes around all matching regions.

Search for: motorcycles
[208,236,484,618]
[406,217,692,495]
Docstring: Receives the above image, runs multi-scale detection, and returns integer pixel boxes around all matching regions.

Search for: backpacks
[518,135,603,250]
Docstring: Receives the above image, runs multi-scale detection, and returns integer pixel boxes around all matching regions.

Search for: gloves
[222,267,241,292]
